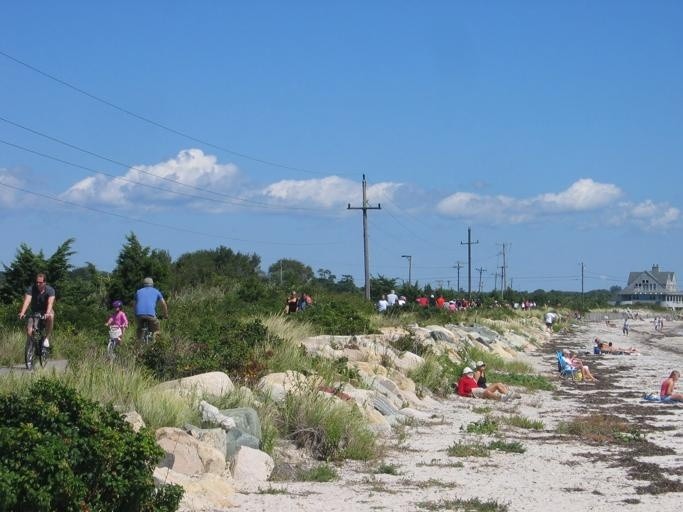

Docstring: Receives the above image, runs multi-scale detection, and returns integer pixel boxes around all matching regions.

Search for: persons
[568,349,600,383]
[560,348,584,384]
[302,293,313,305]
[376,288,538,315]
[286,290,298,314]
[455,366,522,402]
[472,360,514,397]
[593,337,640,356]
[621,318,630,337]
[133,276,168,343]
[653,317,658,331]
[16,272,56,348]
[294,292,309,311]
[657,317,664,333]
[545,312,555,333]
[102,299,129,341]
[660,370,683,403]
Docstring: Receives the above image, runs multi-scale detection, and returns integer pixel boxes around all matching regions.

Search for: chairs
[554,349,578,385]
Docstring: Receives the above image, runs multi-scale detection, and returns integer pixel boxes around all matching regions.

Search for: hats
[463,366,473,374]
[477,360,485,366]
[143,277,153,285]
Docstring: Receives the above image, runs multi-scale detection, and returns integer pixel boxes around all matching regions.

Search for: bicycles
[102,324,127,360]
[16,311,49,373]
[137,314,167,353]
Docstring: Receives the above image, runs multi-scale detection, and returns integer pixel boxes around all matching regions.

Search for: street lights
[400,254,412,284]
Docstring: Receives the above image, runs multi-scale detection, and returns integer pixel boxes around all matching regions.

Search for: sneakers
[499,390,522,403]
[42,338,50,348]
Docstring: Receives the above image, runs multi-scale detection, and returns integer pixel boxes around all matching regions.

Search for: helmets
[112,299,123,308]
